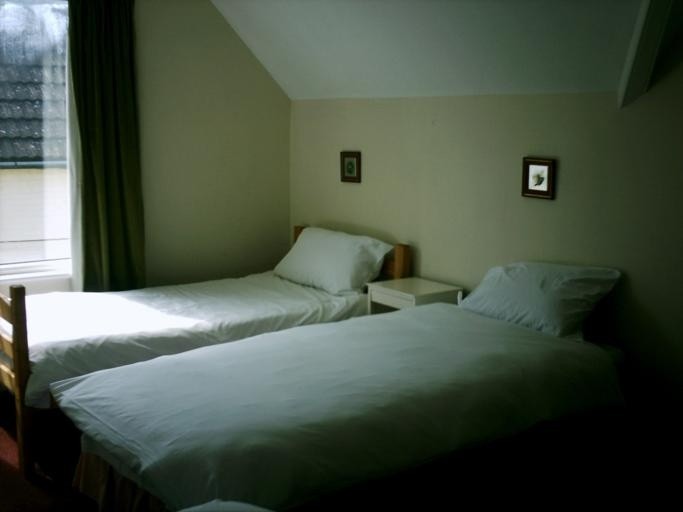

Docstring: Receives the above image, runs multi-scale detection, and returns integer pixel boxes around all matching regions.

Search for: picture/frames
[521,156,558,200]
[339,151,362,182]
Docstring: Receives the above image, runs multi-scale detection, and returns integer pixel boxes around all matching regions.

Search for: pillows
[271,226,395,295]
[460,262,624,338]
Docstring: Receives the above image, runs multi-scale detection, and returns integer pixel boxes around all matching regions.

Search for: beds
[48,291,621,512]
[0,226,410,484]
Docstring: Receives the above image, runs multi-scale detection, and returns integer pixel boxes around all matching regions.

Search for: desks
[364,279,462,311]
